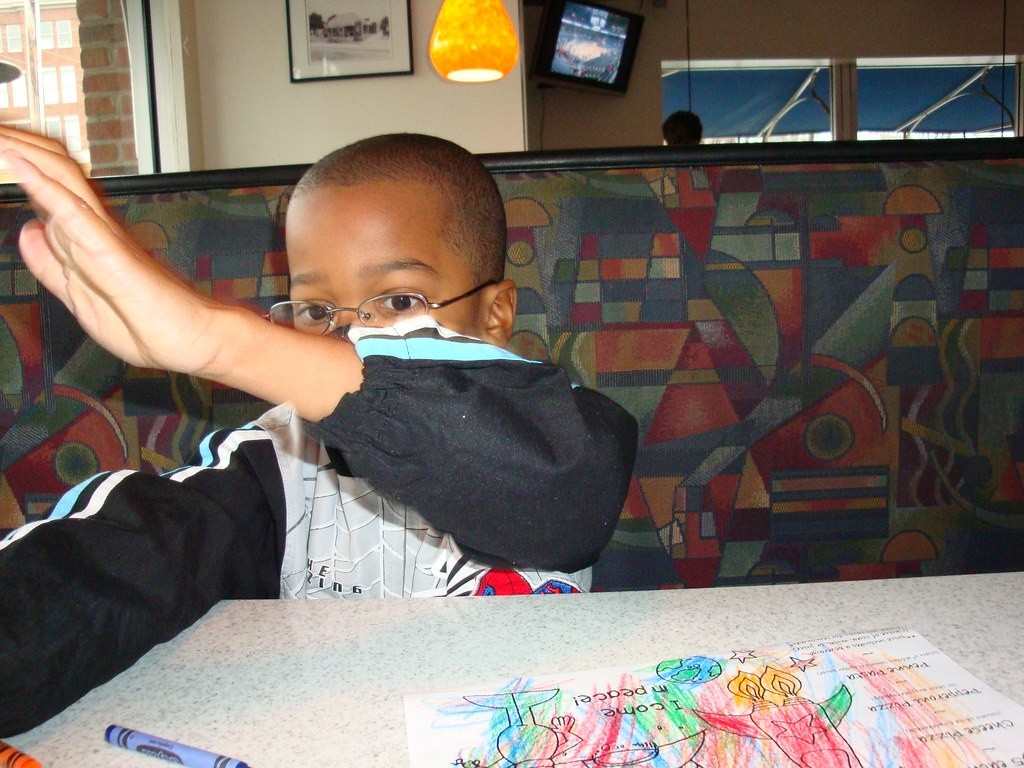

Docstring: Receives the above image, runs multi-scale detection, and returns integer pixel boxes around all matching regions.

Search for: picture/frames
[285,1,414,83]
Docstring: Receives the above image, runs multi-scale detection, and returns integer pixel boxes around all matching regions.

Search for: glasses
[262,279,498,336]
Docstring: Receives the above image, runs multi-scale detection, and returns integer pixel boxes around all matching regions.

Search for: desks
[0,571,1024,768]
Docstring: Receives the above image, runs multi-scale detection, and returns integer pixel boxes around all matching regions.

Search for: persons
[0,124,639,739]
[664,110,702,145]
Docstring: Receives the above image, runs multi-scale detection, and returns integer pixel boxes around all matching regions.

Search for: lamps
[428,0,520,83]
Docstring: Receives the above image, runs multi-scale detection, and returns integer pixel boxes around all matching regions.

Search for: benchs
[0,137,1024,593]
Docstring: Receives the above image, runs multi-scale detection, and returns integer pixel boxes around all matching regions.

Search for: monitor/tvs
[528,0,645,96]
